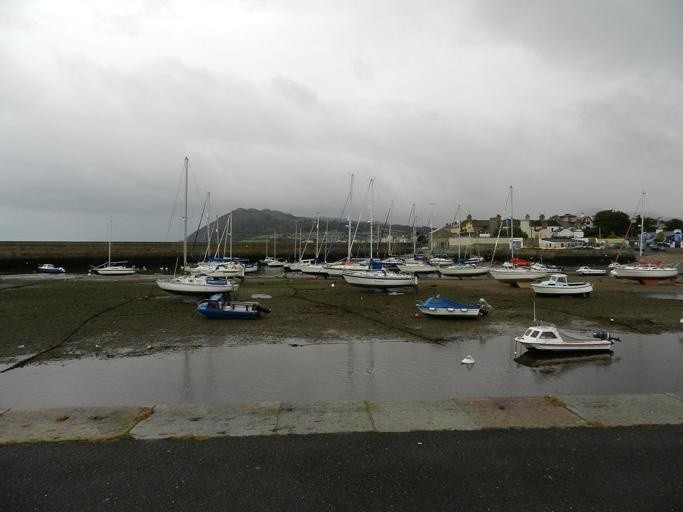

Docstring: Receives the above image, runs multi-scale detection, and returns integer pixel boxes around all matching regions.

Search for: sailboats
[88,216,136,275]
[610,190,678,280]
[489,184,548,280]
[259,232,287,267]
[284,173,489,287]
[154,156,257,294]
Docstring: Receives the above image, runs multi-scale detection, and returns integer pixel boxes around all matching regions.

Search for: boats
[515,322,622,358]
[416,294,491,319]
[530,273,593,300]
[37,263,66,273]
[196,292,271,319]
[575,266,606,275]
